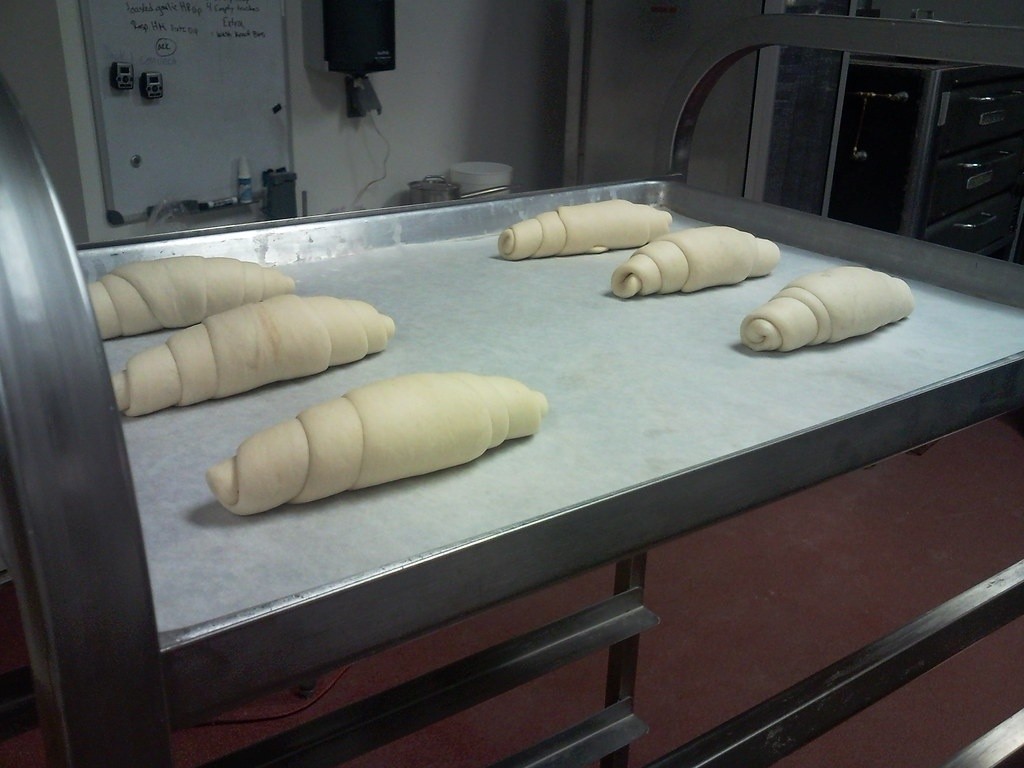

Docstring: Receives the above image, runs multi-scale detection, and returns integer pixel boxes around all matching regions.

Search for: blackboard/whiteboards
[77,1,299,226]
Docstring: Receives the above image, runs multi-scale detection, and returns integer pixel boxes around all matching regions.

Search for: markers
[262,170,269,210]
[200,196,238,210]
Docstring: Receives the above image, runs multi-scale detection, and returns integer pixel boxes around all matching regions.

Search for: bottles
[237,155,253,203]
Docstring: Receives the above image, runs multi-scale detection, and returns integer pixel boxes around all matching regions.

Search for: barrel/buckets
[449,161,513,199]
[408,175,459,205]
